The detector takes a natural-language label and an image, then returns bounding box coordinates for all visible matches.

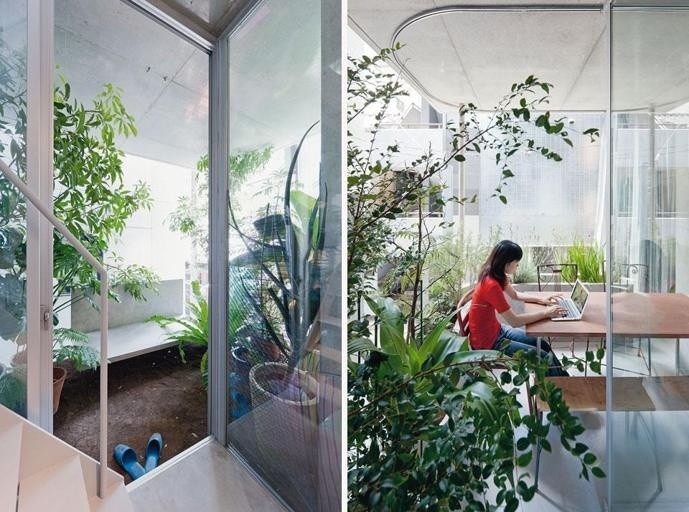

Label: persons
[468,240,570,377]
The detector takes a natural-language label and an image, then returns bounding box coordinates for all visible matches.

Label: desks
[524,291,689,492]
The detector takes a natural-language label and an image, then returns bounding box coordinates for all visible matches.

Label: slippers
[143,431,163,472]
[113,444,144,480]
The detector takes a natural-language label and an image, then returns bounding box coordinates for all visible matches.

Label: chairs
[457,288,533,416]
[536,261,652,376]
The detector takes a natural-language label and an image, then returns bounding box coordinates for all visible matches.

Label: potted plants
[224,118,328,471]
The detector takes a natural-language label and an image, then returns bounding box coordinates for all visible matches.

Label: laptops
[546,278,589,321]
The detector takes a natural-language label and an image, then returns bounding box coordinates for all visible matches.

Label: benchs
[532,376,689,491]
